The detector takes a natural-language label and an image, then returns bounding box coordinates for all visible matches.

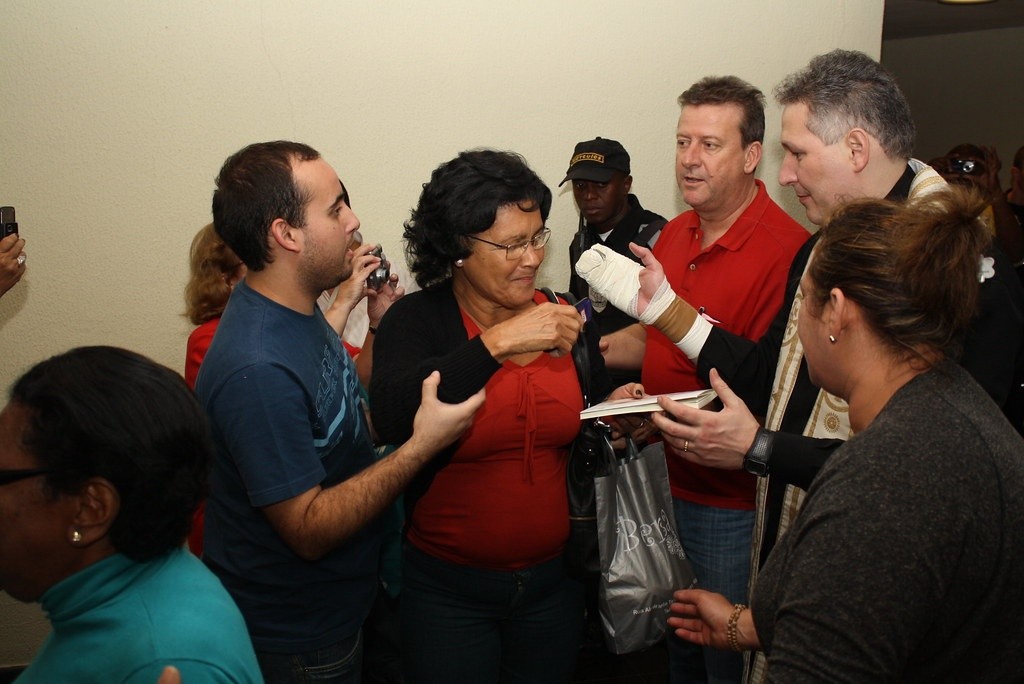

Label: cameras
[951,158,978,175]
[363,244,390,293]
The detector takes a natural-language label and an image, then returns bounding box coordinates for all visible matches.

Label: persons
[368,147,653,683]
[929,143,1024,440]
[668,174,1024,684]
[185,141,487,683]
[0,346,264,683]
[0,204,28,304]
[556,48,990,684]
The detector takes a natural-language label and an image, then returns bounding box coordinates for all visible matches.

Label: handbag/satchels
[594,424,699,654]
[544,288,619,583]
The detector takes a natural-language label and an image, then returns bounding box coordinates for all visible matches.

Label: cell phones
[573,297,592,326]
[0,206,18,242]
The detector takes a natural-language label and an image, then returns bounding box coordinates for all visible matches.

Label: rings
[635,419,646,428]
[684,439,688,451]
[17,255,26,266]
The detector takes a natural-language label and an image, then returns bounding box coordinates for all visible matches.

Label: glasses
[469,226,551,260]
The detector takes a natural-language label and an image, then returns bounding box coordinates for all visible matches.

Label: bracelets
[368,325,377,334]
[727,604,746,652]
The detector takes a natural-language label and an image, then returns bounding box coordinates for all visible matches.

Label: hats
[558,137,631,187]
